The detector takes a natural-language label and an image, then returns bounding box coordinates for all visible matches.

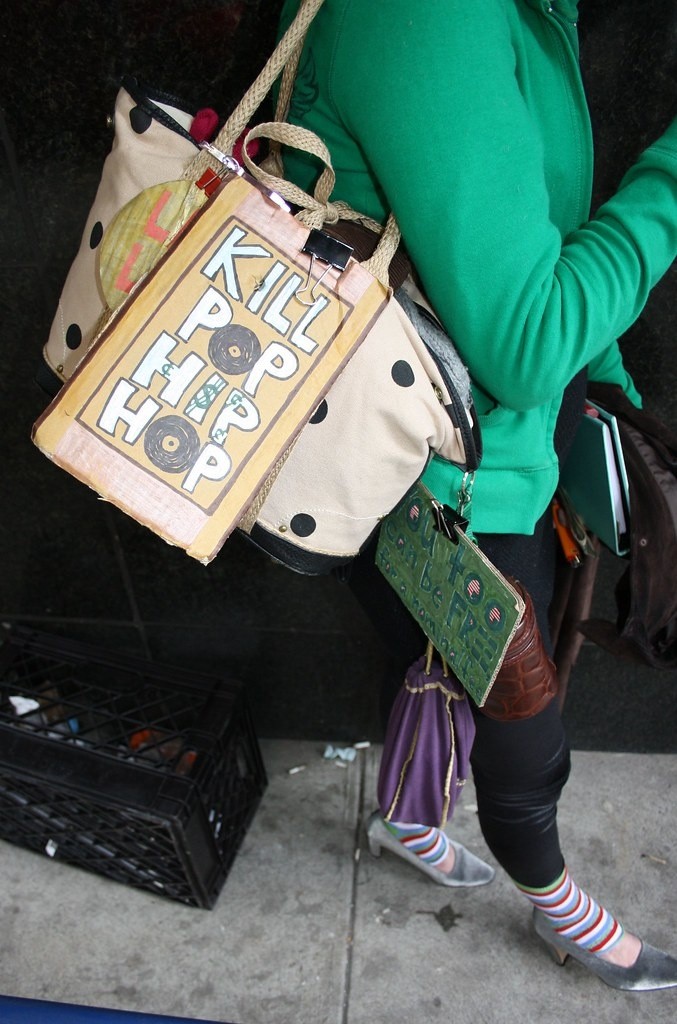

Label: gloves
[187,108,259,169]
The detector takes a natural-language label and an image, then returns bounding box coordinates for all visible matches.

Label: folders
[556,396,637,556]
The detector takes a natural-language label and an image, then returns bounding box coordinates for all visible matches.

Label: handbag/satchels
[43,1,483,577]
[376,636,476,834]
[477,573,560,724]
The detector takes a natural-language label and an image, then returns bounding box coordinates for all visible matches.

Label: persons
[274,0,677,993]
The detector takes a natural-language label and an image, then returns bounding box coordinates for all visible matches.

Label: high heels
[531,905,677,992]
[365,809,497,888]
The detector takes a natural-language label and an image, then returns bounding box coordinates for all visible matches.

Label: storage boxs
[0,623,266,907]
